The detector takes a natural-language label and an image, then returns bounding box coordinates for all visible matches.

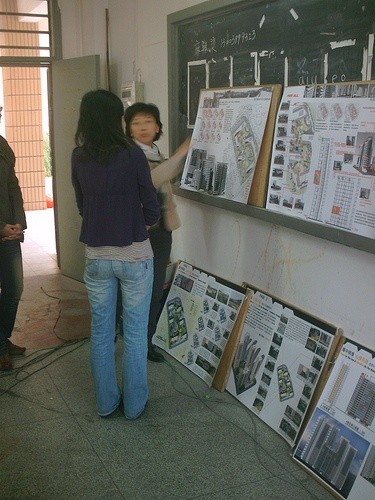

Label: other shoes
[0,350,12,371]
[147,348,165,362]
[5,342,26,355]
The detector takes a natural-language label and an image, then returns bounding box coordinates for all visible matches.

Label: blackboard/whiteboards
[164,1,375,254]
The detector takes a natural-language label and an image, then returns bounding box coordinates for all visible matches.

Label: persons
[71,89,161,420]
[124,102,190,362]
[0,135,27,371]
